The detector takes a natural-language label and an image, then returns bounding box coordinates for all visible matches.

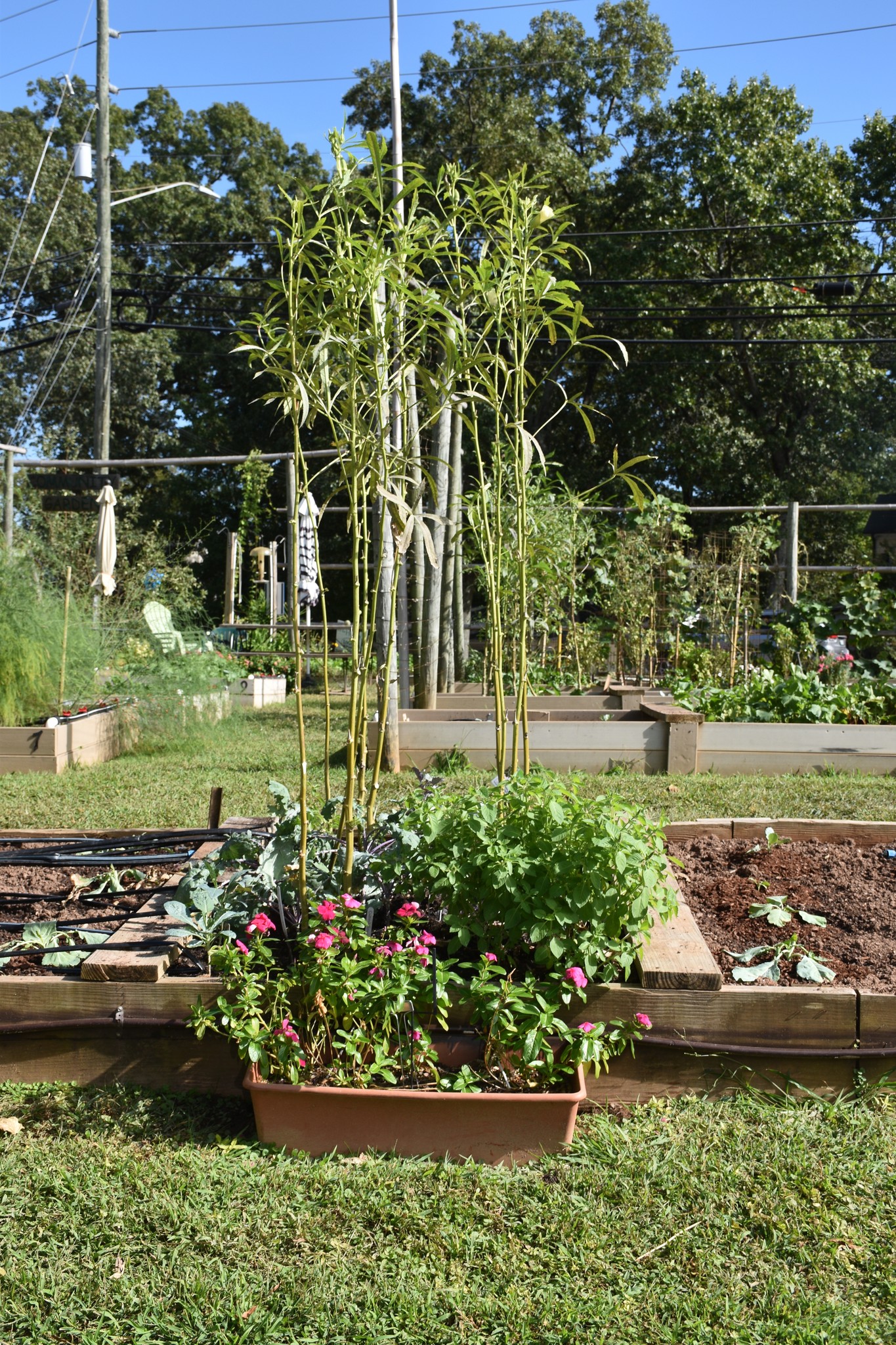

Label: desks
[220,623,372,694]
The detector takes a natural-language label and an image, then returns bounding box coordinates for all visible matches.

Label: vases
[237,1036,596,1157]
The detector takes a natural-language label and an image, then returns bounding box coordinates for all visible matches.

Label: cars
[685,610,850,658]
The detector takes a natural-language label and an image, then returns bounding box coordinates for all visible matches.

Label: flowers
[185,891,649,1094]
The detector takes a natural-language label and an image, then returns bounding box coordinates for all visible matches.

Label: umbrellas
[297,492,320,674]
[90,479,117,623]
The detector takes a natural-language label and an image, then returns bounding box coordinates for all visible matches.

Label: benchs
[231,650,377,659]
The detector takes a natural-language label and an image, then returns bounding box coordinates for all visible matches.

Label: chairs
[145,602,212,662]
